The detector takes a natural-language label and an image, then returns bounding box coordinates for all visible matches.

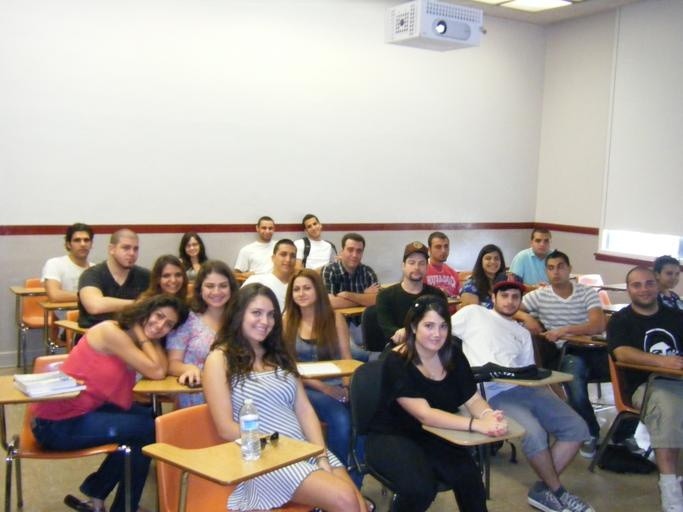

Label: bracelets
[466,416,476,433]
[478,407,494,420]
[136,338,151,350]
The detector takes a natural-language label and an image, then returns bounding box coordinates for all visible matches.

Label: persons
[518,249,612,460]
[509,225,555,287]
[175,231,208,283]
[450,272,595,512]
[279,268,366,492]
[292,214,336,276]
[459,244,524,311]
[71,228,153,351]
[203,283,368,512]
[163,261,238,388]
[374,241,449,350]
[366,294,509,512]
[232,217,279,280]
[605,267,683,512]
[236,239,297,317]
[136,254,195,306]
[317,232,380,347]
[423,231,463,315]
[48,222,93,346]
[47,294,191,511]
[653,255,683,310]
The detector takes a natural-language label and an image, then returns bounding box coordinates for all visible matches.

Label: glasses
[259,431,280,447]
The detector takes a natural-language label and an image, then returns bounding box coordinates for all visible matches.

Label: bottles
[238,399,261,460]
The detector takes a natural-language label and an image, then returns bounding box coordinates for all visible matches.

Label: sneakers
[525,488,571,511]
[579,437,597,457]
[617,438,645,455]
[558,491,595,512]
[657,479,683,512]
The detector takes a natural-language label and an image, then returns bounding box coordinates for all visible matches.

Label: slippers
[63,494,107,512]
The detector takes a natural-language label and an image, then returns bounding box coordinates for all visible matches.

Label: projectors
[386,1,484,52]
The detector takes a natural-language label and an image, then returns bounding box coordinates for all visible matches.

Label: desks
[0,373,84,451]
[38,301,78,355]
[9,283,47,326]
[335,305,370,319]
[54,320,93,351]
[595,281,634,292]
[142,432,325,511]
[490,363,575,389]
[603,303,628,312]
[566,273,579,280]
[295,357,365,382]
[421,409,526,447]
[133,377,205,416]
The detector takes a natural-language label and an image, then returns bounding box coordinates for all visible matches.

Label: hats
[403,242,429,262]
[493,272,528,293]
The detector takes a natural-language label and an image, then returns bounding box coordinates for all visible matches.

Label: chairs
[588,351,656,472]
[349,358,493,509]
[53,303,70,347]
[150,401,316,512]
[17,274,60,368]
[65,307,83,353]
[3,352,133,512]
[532,318,618,402]
